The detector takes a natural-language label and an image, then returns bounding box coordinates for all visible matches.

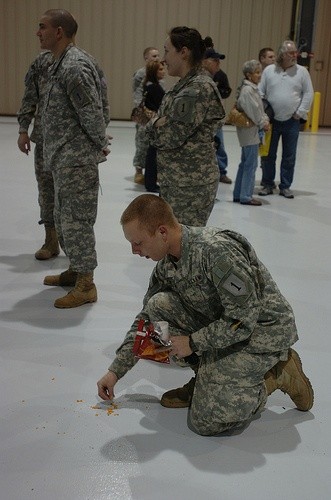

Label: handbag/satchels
[258,122,272,156]
[229,84,254,128]
[130,91,154,126]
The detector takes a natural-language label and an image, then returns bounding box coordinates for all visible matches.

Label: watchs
[188,334,200,352]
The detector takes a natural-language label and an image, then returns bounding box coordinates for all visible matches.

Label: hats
[203,47,225,60]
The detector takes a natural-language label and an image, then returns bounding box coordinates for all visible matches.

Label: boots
[55,272,97,307]
[135,167,144,185]
[35,223,60,259]
[160,362,199,408]
[44,265,78,287]
[263,348,314,411]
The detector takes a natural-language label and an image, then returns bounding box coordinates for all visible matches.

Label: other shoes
[146,184,161,193]
[261,182,276,189]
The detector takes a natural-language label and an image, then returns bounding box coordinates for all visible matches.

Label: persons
[36,10,114,308]
[17,9,111,260]
[97,194,314,436]
[128,26,315,228]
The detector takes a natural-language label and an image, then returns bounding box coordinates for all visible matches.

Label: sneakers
[259,184,273,195]
[279,188,294,198]
[220,176,232,184]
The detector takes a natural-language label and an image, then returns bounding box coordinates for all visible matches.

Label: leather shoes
[233,198,262,206]
